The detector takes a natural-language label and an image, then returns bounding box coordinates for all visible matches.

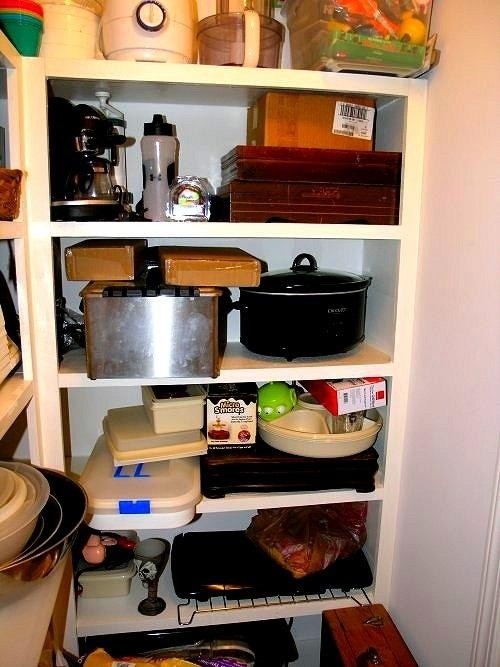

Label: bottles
[140,114,177,221]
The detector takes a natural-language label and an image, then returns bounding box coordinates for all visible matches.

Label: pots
[232,252,374,363]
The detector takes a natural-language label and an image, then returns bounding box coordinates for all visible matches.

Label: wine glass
[134,538,171,616]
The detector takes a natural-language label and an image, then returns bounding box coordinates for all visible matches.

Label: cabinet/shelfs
[0,0,429,667]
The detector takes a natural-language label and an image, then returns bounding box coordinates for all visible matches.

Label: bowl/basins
[257,417,382,458]
[0,0,41,55]
[0,460,89,608]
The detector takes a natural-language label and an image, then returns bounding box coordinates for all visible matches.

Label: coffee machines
[48,98,122,221]
